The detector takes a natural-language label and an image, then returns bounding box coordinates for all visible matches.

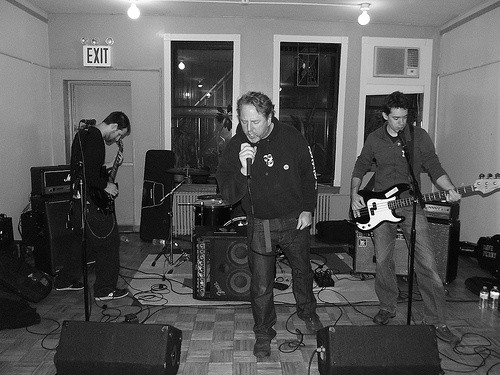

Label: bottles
[490,286,500,310]
[479,285,489,310]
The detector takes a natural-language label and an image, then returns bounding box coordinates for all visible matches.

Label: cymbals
[165,168,211,177]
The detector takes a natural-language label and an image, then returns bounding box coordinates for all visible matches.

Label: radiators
[171,191,331,238]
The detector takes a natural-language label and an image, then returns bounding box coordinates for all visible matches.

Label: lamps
[78,36,113,67]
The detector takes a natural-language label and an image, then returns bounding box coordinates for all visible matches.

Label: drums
[193,199,233,229]
[223,216,247,227]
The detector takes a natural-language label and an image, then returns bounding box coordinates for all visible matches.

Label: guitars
[79,137,124,241]
[351,173,500,234]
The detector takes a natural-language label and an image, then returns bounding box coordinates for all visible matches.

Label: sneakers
[297,311,324,333]
[94,288,130,300]
[55,282,84,290]
[435,325,455,342]
[254,337,271,357]
[373,310,396,324]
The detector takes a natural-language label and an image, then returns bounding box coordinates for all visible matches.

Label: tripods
[150,176,190,267]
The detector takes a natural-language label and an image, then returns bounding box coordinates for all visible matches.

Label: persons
[217,91,323,357]
[55,110,131,301]
[351,91,461,344]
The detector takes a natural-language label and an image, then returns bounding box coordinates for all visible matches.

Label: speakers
[30,199,90,271]
[426,220,460,282]
[54,321,181,375]
[192,236,253,302]
[316,324,443,375]
[353,235,411,277]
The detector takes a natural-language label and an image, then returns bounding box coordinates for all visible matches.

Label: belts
[263,219,272,253]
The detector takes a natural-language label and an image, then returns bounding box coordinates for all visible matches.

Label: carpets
[126,253,380,307]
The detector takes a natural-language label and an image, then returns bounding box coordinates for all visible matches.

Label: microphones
[246,158,253,176]
[397,130,408,148]
[80,119,97,125]
